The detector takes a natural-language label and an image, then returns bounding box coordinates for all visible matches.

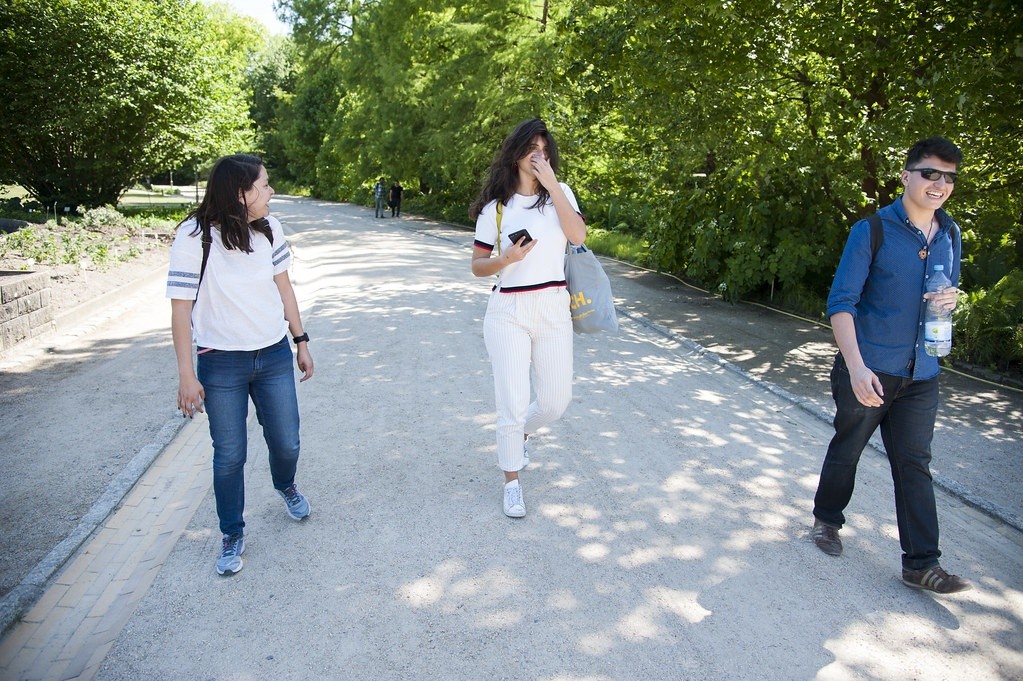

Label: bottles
[924,265,951,357]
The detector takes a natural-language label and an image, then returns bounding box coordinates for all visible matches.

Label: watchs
[293,332,310,344]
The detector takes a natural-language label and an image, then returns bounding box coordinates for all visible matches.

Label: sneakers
[278,484,311,520]
[813,517,843,556]
[216,534,245,574]
[523,436,529,465]
[503,479,526,517]
[902,564,973,594]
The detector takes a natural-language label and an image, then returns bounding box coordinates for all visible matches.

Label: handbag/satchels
[564,241,618,334]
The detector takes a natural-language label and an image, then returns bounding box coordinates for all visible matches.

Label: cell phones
[507,229,532,247]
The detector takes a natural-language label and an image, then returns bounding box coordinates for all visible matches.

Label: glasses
[908,169,959,184]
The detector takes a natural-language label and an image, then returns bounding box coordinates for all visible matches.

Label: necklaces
[910,217,933,260]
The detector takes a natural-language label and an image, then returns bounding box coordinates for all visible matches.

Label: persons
[166,154,314,576]
[812,137,973,594]
[374,177,386,218]
[472,118,586,518]
[390,180,403,217]
